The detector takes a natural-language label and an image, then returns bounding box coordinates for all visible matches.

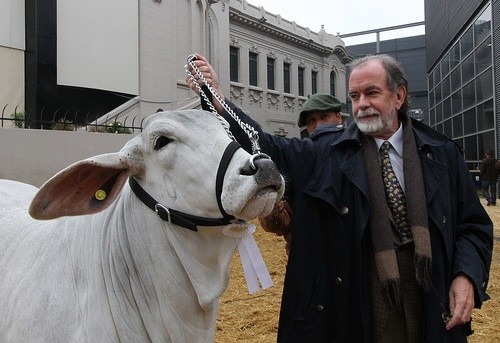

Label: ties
[379,140,413,240]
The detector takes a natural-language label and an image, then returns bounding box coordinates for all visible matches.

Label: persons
[188,54,493,343]
[479,150,498,205]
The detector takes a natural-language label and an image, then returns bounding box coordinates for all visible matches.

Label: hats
[299,94,342,128]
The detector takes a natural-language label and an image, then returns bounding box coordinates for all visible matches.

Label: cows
[0,108,286,343]
